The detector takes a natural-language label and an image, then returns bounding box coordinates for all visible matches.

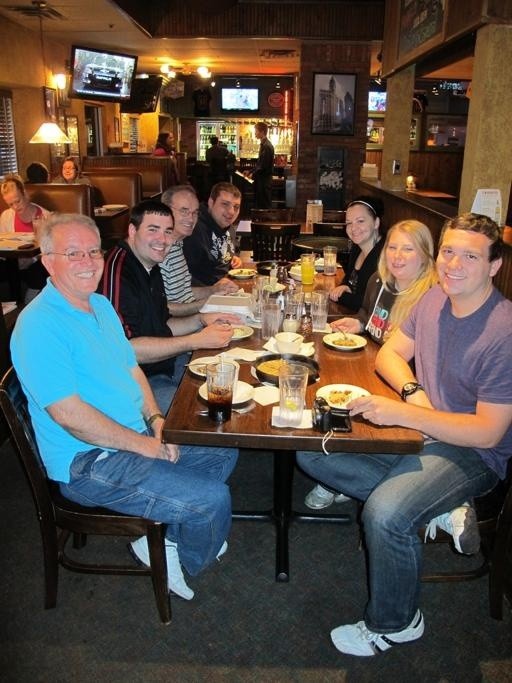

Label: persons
[156,183,239,314]
[295,213,511,656]
[52,156,95,207]
[303,221,440,509]
[96,198,245,419]
[9,213,240,600]
[242,122,274,210]
[0,173,51,314]
[26,162,48,184]
[329,196,388,312]
[151,131,181,186]
[183,183,241,287]
[206,137,235,184]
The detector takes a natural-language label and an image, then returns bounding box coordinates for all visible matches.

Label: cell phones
[329,404,352,434]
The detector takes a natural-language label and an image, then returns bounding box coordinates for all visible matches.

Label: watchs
[401,382,422,402]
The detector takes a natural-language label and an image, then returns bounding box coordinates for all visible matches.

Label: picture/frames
[317,145,346,208]
[311,72,356,135]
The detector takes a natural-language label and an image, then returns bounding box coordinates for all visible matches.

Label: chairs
[312,222,349,237]
[239,220,301,261]
[0,364,175,626]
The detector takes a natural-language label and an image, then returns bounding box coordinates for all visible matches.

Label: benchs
[0,165,167,248]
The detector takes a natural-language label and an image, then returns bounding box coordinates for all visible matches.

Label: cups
[311,291,328,328]
[324,246,338,275]
[251,285,268,324]
[261,298,281,338]
[278,364,309,423]
[301,254,315,284]
[208,362,236,423]
[267,332,316,360]
[282,290,305,333]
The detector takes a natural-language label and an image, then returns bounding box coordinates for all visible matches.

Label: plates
[253,354,323,386]
[230,326,254,341]
[198,380,254,405]
[324,333,366,351]
[230,269,256,279]
[315,384,369,410]
[104,204,128,210]
[190,356,240,378]
[19,233,34,241]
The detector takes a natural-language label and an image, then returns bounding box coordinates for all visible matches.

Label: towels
[249,382,282,405]
[271,409,313,428]
[215,346,271,362]
[264,336,278,353]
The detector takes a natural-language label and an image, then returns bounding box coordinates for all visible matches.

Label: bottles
[271,263,287,283]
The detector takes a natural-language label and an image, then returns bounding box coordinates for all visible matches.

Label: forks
[194,402,255,417]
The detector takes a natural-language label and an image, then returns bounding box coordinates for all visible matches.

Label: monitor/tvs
[118,76,163,114]
[65,45,139,104]
[220,85,260,113]
[367,89,387,114]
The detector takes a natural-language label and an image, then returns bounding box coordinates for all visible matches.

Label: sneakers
[212,540,229,563]
[420,501,481,560]
[329,609,424,658]
[335,492,351,505]
[129,528,194,602]
[305,484,334,510]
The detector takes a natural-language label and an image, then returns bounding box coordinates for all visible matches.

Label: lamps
[27,13,74,145]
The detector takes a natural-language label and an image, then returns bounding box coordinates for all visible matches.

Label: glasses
[62,167,76,170]
[169,209,201,219]
[47,249,105,262]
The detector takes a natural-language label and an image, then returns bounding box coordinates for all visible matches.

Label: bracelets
[146,414,163,425]
